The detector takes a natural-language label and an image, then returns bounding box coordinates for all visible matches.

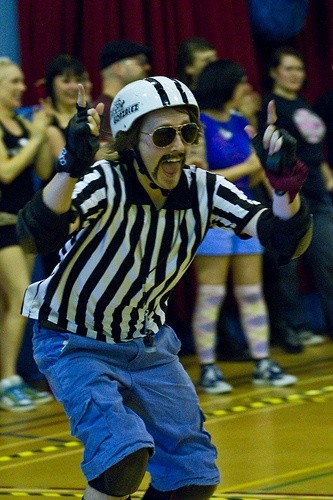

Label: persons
[260,51,333,353]
[187,58,298,394]
[178,37,261,363]
[33,52,93,186]
[15,76,313,500]
[0,55,54,412]
[94,41,152,161]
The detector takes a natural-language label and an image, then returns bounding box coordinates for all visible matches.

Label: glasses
[138,122,200,147]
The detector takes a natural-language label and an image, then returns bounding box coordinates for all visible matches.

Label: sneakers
[196,367,233,393]
[1,382,34,411]
[252,360,298,387]
[20,384,54,405]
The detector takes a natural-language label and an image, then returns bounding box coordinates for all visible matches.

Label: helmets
[111,75,201,140]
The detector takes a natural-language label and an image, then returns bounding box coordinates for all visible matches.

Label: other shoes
[299,331,326,346]
[275,323,303,352]
